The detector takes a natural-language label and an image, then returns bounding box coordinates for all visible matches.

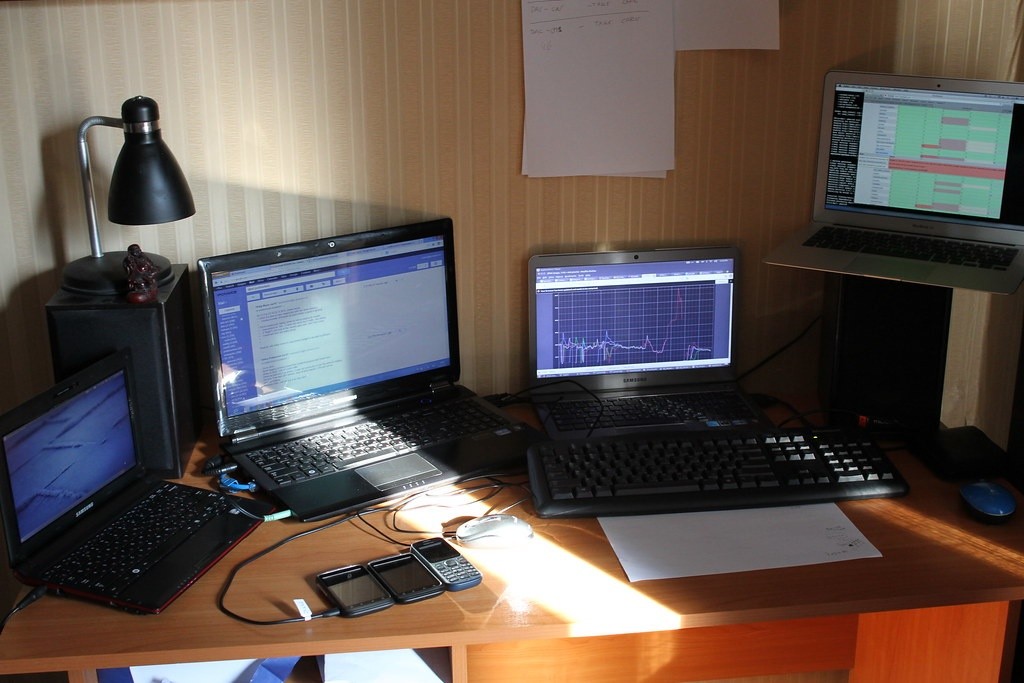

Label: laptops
[0,347,276,617]
[525,246,776,443]
[198,217,550,523]
[759,69,1024,296]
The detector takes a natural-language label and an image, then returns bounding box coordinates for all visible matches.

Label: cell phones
[316,564,395,617]
[368,553,444,605]
[411,537,482,592]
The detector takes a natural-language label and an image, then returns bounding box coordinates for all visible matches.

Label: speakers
[43,264,205,480]
[826,274,952,439]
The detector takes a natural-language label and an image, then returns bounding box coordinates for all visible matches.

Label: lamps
[58,95,196,300]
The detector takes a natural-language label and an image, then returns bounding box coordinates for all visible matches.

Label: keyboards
[526,425,910,518]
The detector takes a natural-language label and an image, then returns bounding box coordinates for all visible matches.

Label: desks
[0,406,1024,683]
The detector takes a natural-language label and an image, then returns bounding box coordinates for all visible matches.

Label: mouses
[455,513,536,545]
[961,480,1017,525]
